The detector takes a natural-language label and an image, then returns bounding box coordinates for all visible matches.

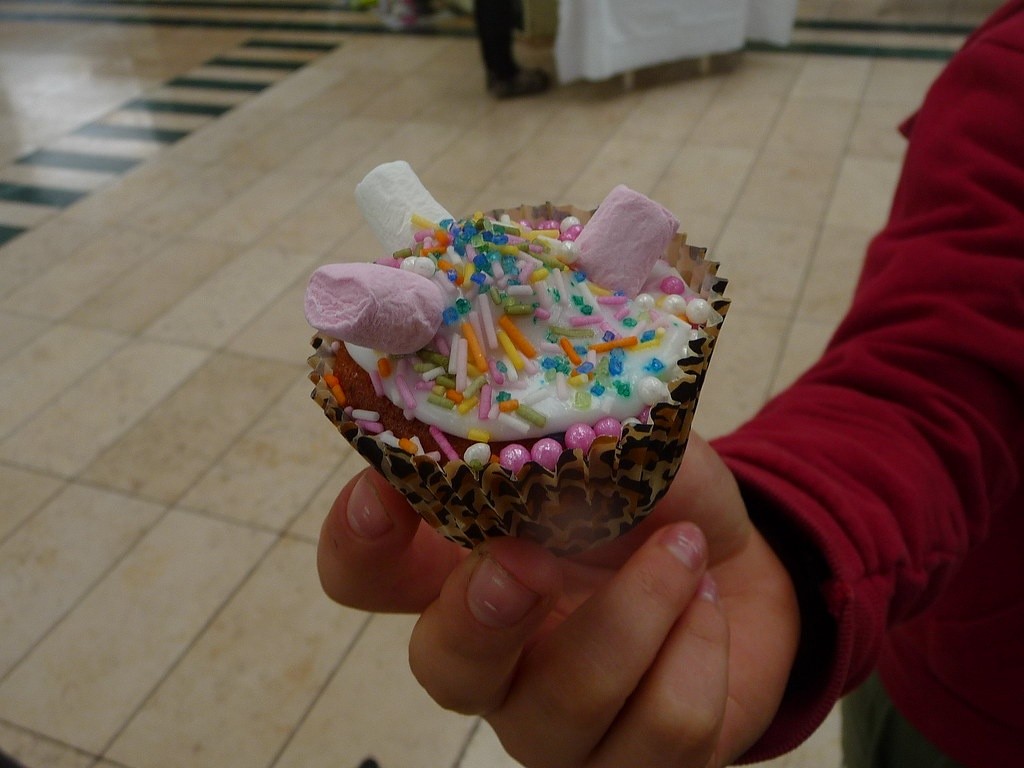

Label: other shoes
[484,68,548,97]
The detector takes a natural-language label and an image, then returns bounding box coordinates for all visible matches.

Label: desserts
[300,159,733,567]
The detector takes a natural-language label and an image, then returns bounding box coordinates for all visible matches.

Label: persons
[316,1,1024,768]
